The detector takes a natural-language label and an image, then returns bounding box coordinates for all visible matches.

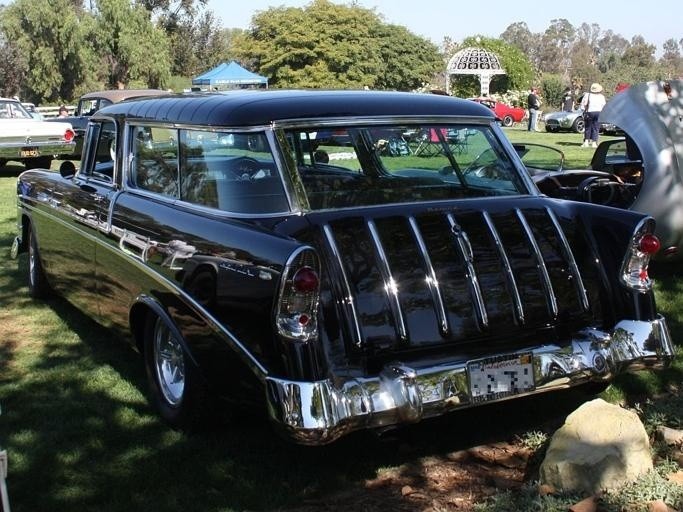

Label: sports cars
[379,77,682,269]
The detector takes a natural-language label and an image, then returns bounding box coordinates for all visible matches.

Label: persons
[580,82,606,148]
[58,107,68,119]
[528,87,540,132]
[561,87,575,113]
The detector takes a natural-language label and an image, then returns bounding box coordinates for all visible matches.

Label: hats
[590,83,602,93]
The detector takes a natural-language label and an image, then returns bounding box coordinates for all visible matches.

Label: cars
[10,86,681,461]
[544,137,644,181]
[0,95,77,173]
